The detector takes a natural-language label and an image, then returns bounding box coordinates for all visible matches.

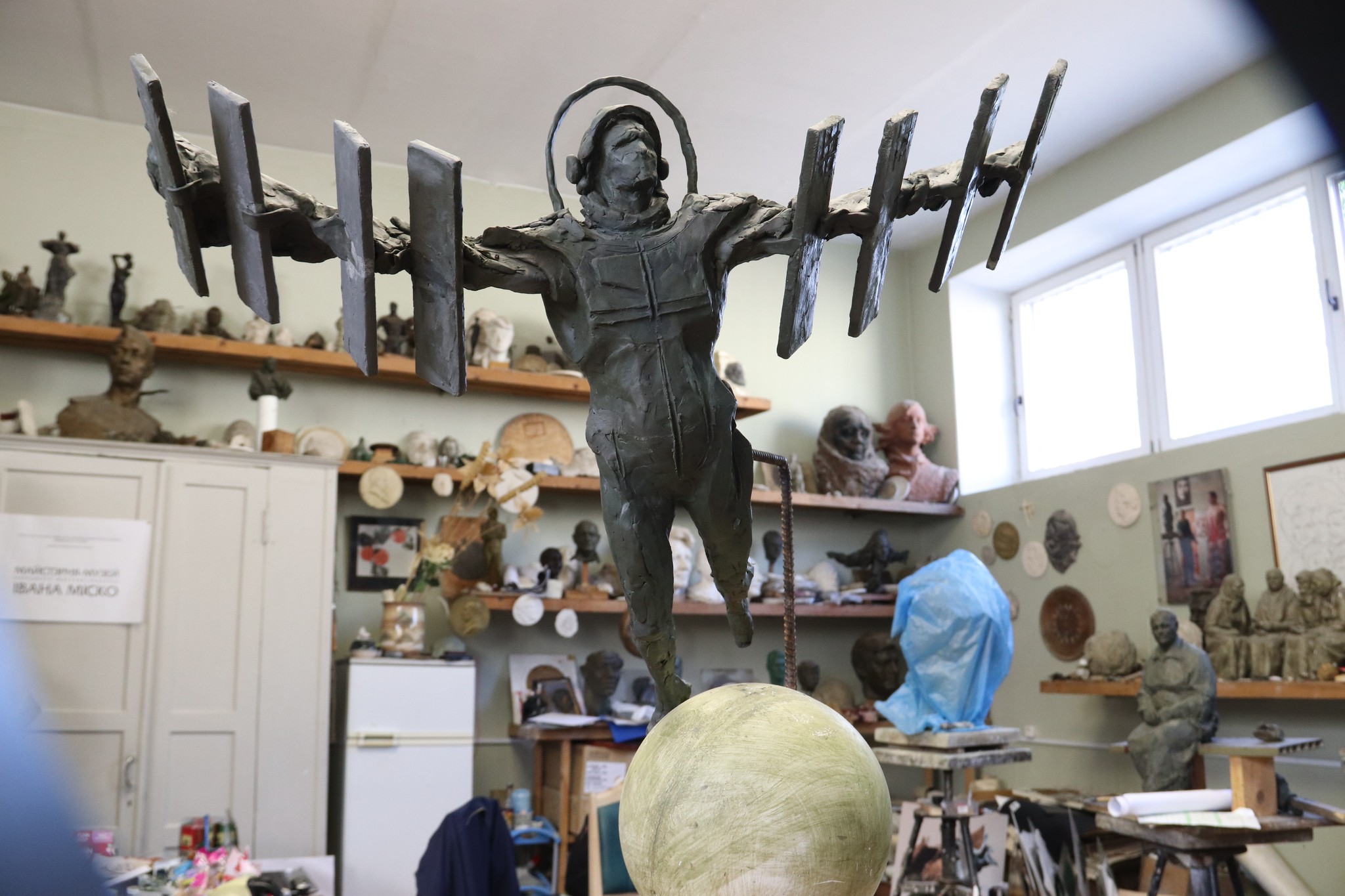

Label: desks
[508,676,1345,896]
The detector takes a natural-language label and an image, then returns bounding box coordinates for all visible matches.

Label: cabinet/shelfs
[0,312,965,616]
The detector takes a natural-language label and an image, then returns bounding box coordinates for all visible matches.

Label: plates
[293,426,350,462]
[495,413,574,469]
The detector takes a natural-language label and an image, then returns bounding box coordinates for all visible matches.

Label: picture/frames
[347,515,424,590]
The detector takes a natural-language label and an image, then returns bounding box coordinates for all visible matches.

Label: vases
[380,602,427,660]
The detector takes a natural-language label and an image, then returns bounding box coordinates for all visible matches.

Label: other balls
[618,682,892,896]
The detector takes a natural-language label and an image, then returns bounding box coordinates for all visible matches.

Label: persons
[668,526,693,601]
[851,631,904,722]
[1204,575,1249,681]
[1126,611,1220,793]
[578,651,623,717]
[572,521,600,562]
[376,301,414,354]
[479,506,509,563]
[56,324,161,443]
[812,406,888,496]
[871,399,960,502]
[143,103,1029,737]
[686,546,724,604]
[1249,567,1345,681]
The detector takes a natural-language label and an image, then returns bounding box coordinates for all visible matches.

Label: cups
[381,602,426,659]
[512,788,532,838]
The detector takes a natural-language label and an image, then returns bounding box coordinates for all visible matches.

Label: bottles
[180,814,238,862]
